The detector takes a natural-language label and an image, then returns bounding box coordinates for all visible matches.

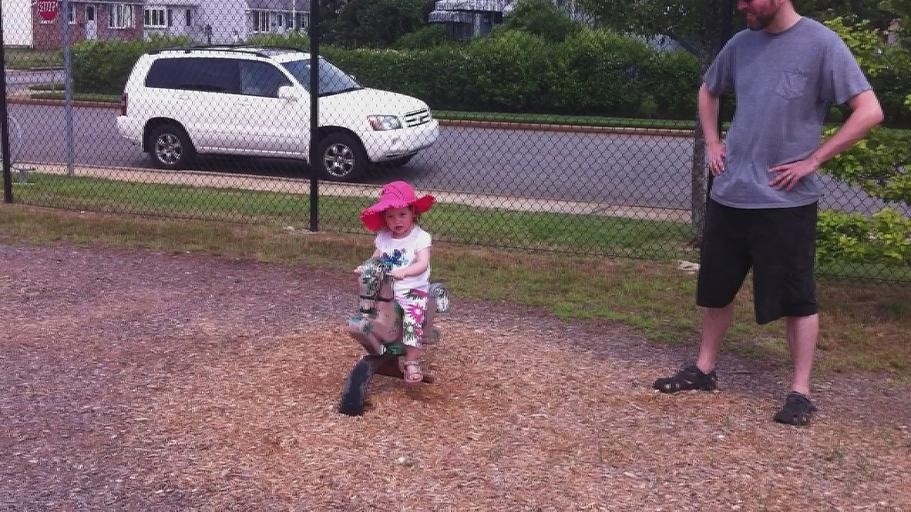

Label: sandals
[773,391,818,425]
[402,357,424,385]
[651,364,717,394]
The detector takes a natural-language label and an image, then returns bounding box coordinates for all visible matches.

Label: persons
[354,181,436,384]
[651,0,885,425]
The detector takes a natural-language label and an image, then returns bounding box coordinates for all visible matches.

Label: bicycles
[0,113,24,172]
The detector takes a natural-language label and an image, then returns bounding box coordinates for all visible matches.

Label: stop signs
[36,0,58,23]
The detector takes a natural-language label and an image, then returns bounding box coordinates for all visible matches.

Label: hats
[360,180,437,233]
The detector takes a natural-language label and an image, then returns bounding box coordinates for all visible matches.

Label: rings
[787,175,792,182]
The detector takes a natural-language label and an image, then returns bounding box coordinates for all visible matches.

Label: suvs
[116,39,438,183]
